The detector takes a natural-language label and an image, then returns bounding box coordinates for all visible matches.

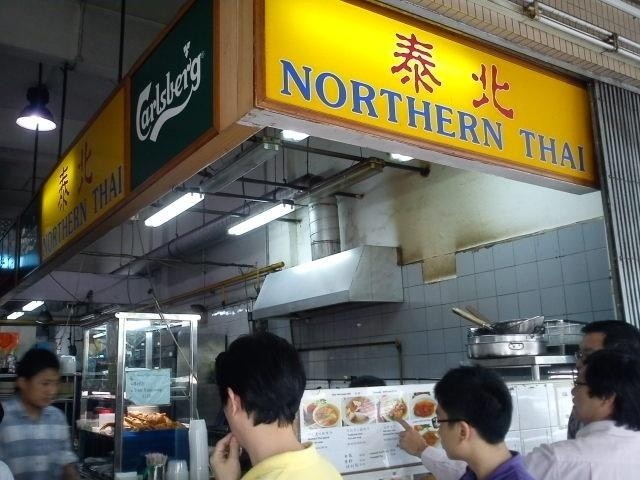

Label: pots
[466,317,586,359]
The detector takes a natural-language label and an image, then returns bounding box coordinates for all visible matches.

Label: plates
[74,310,202,480]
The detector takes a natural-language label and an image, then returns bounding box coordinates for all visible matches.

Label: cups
[432,417,465,429]
[574,351,589,361]
[573,380,589,387]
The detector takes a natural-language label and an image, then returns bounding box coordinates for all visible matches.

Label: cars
[167,459,188,480]
[188,419,210,479]
[142,463,166,479]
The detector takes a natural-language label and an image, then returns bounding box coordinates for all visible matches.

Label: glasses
[15,84,57,133]
[142,136,387,235]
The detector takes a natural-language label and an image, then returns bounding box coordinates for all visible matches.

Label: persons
[0,348,82,480]
[575,320,640,361]
[0,401,14,480]
[567,362,590,438]
[348,377,385,387]
[397,351,640,479]
[432,363,534,480]
[208,329,342,480]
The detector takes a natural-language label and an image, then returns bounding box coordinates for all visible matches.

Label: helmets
[305,394,437,429]
